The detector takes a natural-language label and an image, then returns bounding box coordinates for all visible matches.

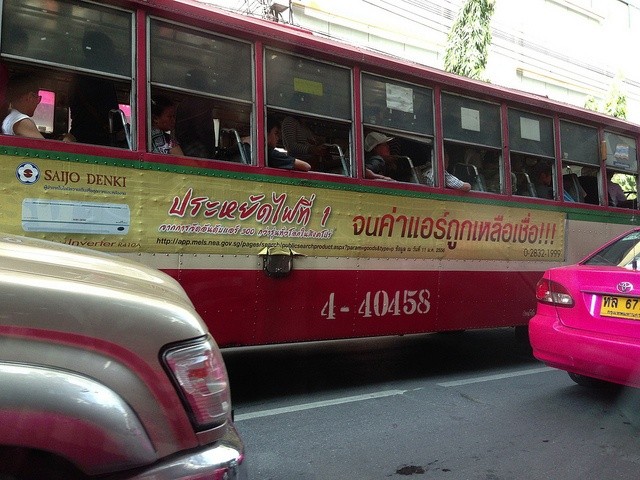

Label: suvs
[1,232,243,480]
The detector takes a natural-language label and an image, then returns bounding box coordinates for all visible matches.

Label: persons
[282,93,330,172]
[268,118,312,171]
[1,25,29,115]
[607,169,628,208]
[2,78,77,142]
[414,141,471,192]
[483,150,517,195]
[151,96,184,155]
[69,31,126,147]
[534,165,565,201]
[238,126,250,146]
[365,131,400,181]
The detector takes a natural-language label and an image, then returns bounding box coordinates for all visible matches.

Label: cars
[527,227,639,403]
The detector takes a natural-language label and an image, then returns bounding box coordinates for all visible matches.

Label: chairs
[517,171,537,197]
[109,108,131,149]
[394,155,423,184]
[325,143,349,176]
[220,127,249,163]
[458,163,485,191]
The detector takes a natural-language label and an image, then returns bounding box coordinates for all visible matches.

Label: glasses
[34,95,41,104]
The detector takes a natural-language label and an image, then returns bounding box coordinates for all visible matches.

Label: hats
[365,132,395,153]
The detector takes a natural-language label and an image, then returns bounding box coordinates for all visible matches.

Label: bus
[1,1,640,355]
